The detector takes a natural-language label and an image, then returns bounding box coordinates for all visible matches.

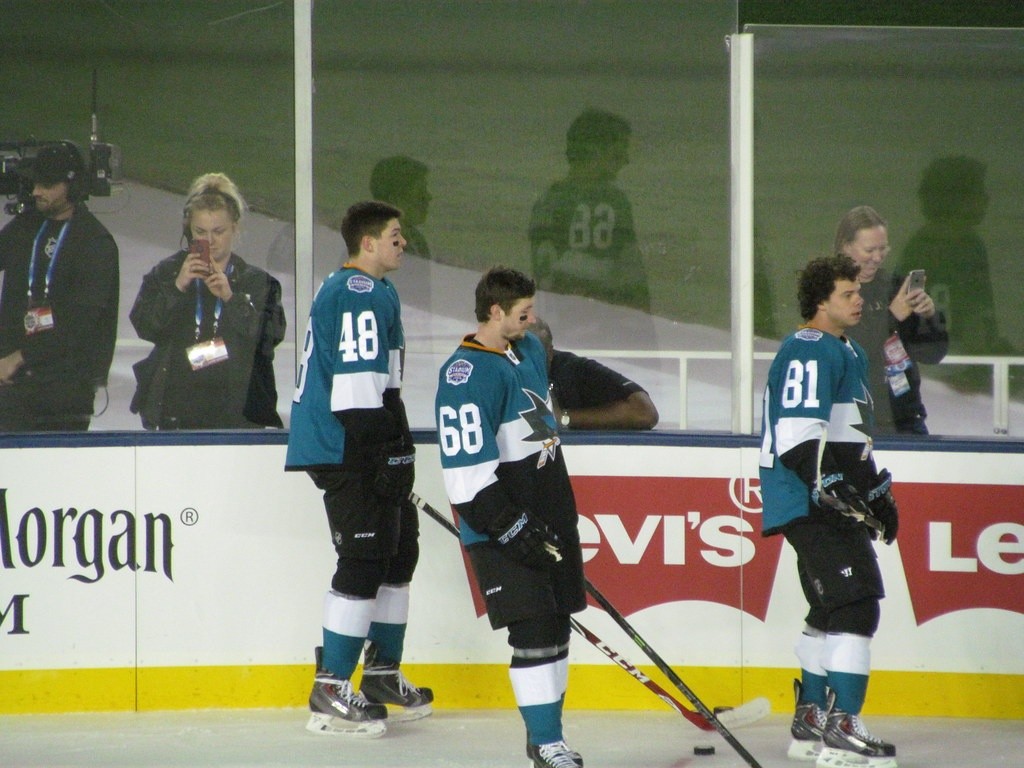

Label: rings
[190,268,192,272]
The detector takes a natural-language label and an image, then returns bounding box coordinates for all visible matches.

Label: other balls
[694,744,715,755]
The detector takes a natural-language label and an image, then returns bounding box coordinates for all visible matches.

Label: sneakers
[819,691,898,767]
[359,638,434,724]
[533,741,584,768]
[307,646,387,737]
[526,733,581,766]
[786,679,828,760]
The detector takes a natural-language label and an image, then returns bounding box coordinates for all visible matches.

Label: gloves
[868,468,898,545]
[376,446,415,507]
[809,471,883,530]
[511,524,570,575]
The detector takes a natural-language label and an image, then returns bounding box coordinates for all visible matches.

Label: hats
[16,141,84,182]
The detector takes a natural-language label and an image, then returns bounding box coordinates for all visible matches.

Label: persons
[284,200,434,739]
[761,255,899,768]
[527,111,661,372]
[893,155,1024,392]
[0,141,120,431]
[527,317,660,431]
[129,172,287,431]
[834,206,948,436]
[434,265,587,768]
[369,157,432,313]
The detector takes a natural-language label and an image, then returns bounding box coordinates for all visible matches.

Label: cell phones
[908,269,926,294]
[191,240,211,276]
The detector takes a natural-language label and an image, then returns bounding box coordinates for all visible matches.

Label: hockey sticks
[812,428,883,533]
[405,489,771,729]
[581,577,762,768]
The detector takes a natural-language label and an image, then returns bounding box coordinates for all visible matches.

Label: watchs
[561,411,570,428]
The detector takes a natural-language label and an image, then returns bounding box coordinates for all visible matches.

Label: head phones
[60,140,91,203]
